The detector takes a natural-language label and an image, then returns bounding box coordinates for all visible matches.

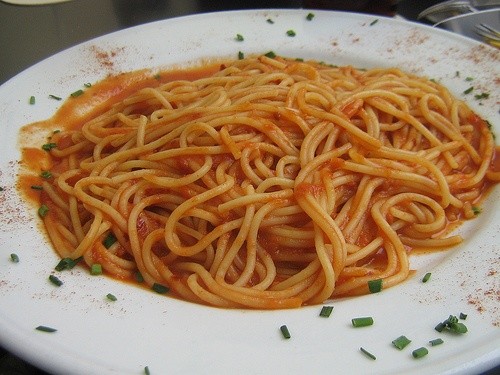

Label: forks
[473,22,500,41]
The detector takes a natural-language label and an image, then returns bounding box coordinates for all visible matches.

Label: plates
[0,9,500,374]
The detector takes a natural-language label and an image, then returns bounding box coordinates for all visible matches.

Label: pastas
[39,57,499,311]
